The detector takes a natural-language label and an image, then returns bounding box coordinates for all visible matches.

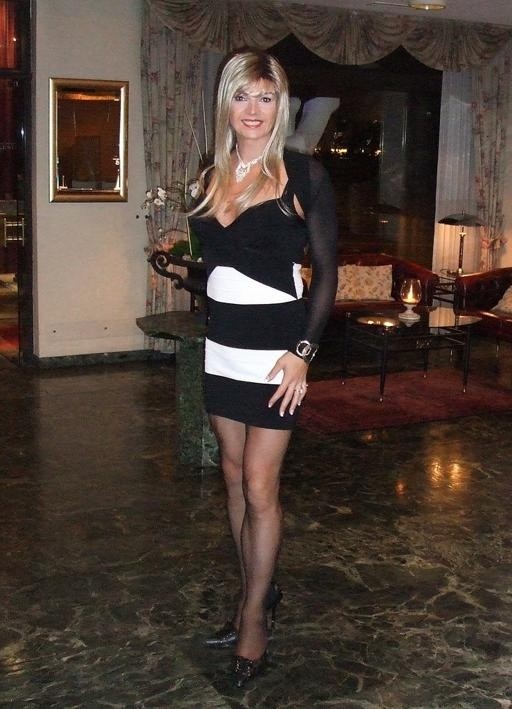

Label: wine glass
[398,276,422,321]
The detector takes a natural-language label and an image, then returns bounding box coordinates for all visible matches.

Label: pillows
[303,262,396,303]
[491,285,511,312]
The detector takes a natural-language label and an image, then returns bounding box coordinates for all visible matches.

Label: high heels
[203,581,284,688]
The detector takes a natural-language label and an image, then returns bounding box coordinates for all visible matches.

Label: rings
[293,389,303,393]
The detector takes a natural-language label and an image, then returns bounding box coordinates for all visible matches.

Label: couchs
[302,250,441,356]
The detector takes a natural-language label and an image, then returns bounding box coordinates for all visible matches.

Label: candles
[402,298,417,303]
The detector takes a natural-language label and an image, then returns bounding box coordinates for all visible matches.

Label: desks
[138,311,222,468]
[431,270,502,359]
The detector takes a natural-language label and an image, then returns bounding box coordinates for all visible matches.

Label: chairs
[454,264,510,353]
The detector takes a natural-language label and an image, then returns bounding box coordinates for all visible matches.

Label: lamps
[437,207,485,274]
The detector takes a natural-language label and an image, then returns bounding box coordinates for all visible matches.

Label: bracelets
[292,338,320,362]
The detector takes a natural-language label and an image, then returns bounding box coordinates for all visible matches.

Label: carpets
[291,367,511,438]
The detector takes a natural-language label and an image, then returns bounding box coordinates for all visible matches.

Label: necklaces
[234,142,272,185]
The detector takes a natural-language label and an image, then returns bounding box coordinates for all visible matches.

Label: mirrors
[47,75,130,202]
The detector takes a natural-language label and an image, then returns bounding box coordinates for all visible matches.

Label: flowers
[141,173,198,220]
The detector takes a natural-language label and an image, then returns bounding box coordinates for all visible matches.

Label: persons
[180,51,340,688]
